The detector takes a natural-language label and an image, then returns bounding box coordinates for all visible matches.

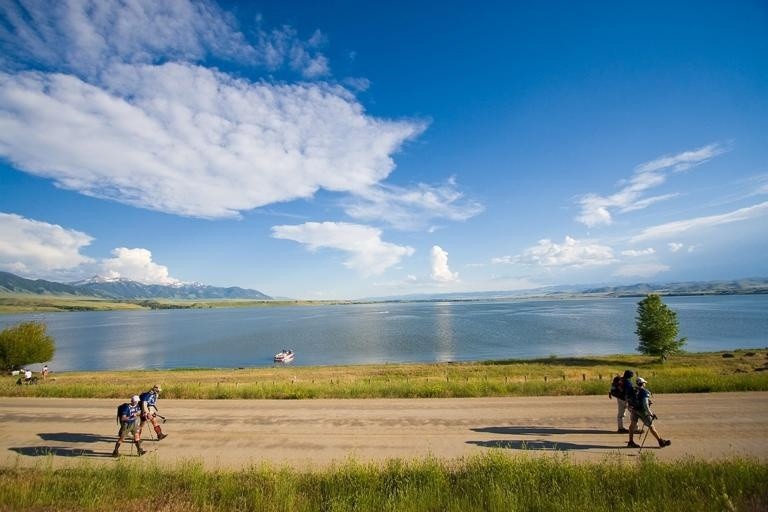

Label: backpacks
[628,387,646,411]
[611,376,630,398]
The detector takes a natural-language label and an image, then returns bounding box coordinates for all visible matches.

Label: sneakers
[618,428,670,448]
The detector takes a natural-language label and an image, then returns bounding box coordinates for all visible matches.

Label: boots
[112,432,168,457]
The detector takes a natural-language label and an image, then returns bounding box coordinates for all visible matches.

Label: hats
[152,384,162,391]
[132,395,141,402]
[635,377,648,385]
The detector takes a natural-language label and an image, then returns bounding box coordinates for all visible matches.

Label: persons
[627,377,671,447]
[42,364,48,379]
[25,369,32,381]
[132,384,168,443]
[112,395,146,457]
[617,371,645,434]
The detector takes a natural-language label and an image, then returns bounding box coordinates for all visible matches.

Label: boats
[274,349,295,364]
